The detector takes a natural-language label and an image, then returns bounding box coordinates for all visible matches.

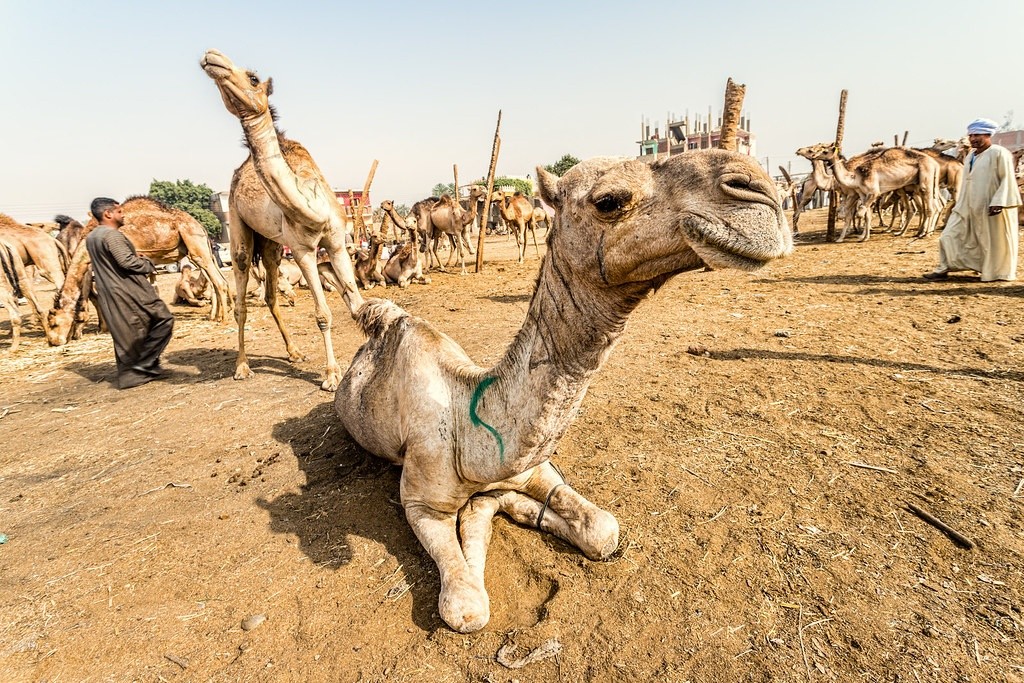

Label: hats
[967,118,1000,137]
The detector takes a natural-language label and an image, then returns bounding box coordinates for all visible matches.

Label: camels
[200,47,365,393]
[792,137,973,244]
[0,186,550,347]
[337,148,796,634]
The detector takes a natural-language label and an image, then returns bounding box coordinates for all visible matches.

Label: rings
[994,209,997,213]
[997,210,1001,213]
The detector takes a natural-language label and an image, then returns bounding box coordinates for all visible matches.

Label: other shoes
[132,364,164,375]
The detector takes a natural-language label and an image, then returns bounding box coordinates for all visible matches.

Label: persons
[923,119,1022,283]
[85,197,174,389]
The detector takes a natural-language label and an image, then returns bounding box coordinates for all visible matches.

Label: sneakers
[922,272,948,280]
